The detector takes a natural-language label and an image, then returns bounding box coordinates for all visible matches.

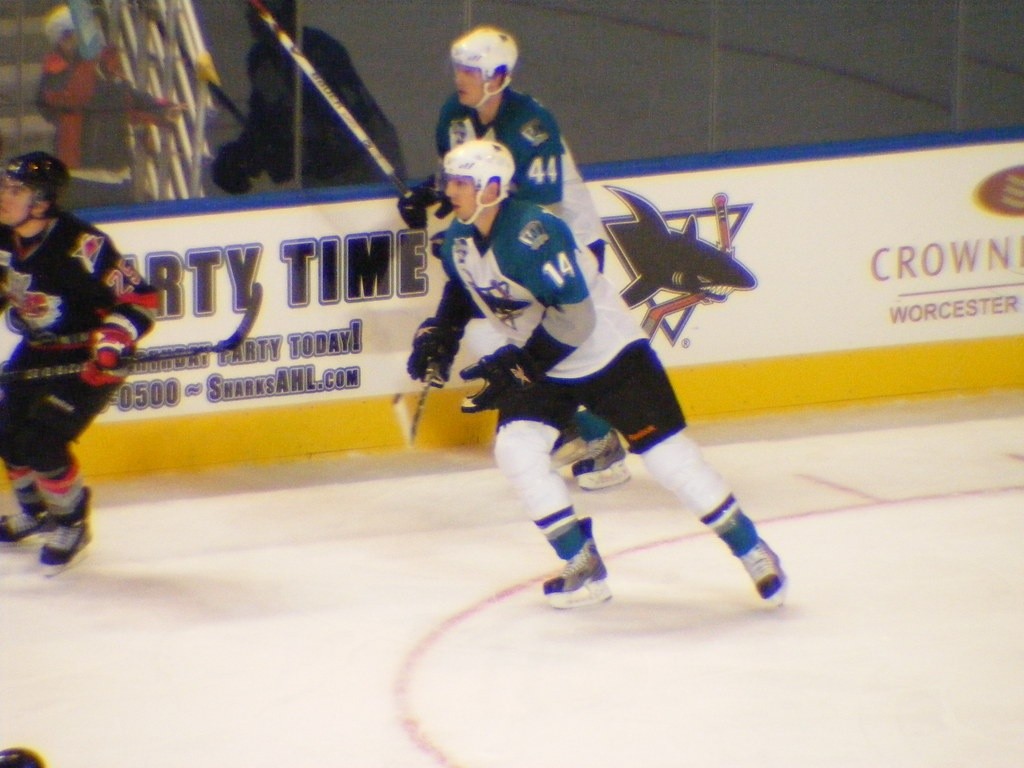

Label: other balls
[0,747,46,768]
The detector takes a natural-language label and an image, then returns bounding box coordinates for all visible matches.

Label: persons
[406,140,785,600]
[34,4,190,208]
[0,150,158,564]
[397,28,626,478]
[211,0,406,196]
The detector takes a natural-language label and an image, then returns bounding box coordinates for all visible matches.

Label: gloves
[210,141,255,194]
[406,316,462,381]
[82,325,137,388]
[458,344,547,414]
[398,181,455,236]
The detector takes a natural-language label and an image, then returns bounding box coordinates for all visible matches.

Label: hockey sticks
[391,360,441,449]
[251,2,413,200]
[1,280,267,388]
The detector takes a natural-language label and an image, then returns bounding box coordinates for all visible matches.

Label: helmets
[443,138,517,201]
[43,8,77,47]
[451,26,518,81]
[6,151,69,206]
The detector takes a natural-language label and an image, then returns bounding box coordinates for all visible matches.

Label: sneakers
[543,516,614,609]
[0,511,53,548]
[572,426,632,489]
[738,537,789,607]
[40,487,94,576]
